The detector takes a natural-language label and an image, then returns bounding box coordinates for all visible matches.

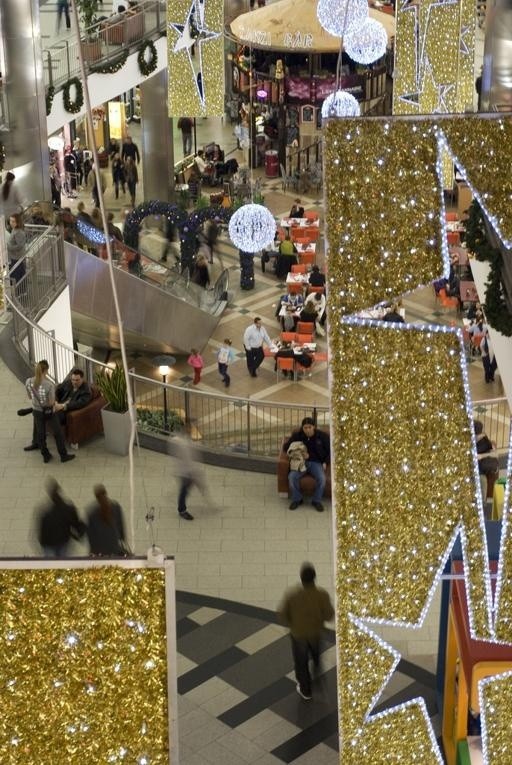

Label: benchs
[100,4,146,46]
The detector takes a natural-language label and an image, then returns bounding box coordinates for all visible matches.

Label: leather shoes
[179,511,194,520]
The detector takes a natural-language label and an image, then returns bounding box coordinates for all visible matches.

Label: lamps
[339,15,393,66]
[227,1,281,260]
[319,1,365,121]
[312,1,371,35]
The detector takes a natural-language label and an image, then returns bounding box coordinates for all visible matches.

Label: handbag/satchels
[42,405,53,419]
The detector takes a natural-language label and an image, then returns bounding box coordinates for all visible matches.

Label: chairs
[43,386,108,448]
[434,209,490,358]
[275,425,332,500]
[259,206,329,387]
[181,137,324,209]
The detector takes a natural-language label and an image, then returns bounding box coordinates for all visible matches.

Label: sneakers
[311,499,324,512]
[23,442,38,451]
[484,375,496,385]
[16,406,33,417]
[289,499,304,511]
[44,454,53,463]
[60,454,76,462]
[296,683,313,701]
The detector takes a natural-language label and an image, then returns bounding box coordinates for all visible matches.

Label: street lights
[126,353,144,405]
[152,355,177,432]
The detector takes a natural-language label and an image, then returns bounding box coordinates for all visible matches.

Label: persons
[94,0,138,27]
[280,562,334,700]
[3,52,511,559]
[55,0,71,31]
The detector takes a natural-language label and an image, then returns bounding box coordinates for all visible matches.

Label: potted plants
[94,360,137,457]
[75,0,104,65]
